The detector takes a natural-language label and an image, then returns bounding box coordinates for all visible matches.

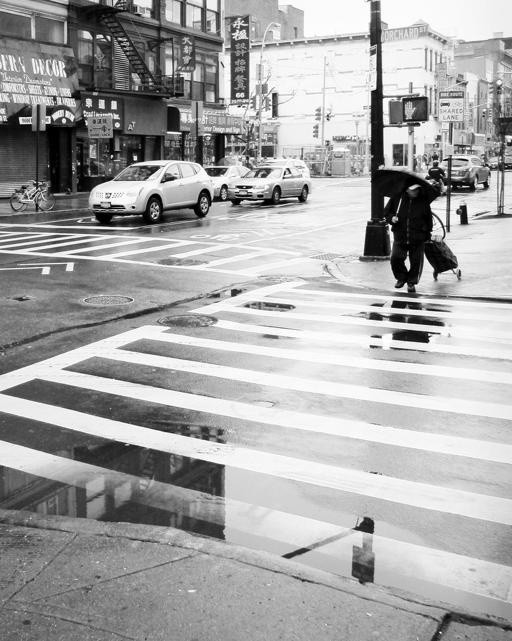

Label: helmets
[433,160,439,165]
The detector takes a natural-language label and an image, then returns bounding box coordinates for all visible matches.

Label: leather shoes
[395,280,406,288]
[407,282,415,292]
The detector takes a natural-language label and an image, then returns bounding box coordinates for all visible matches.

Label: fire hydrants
[457,201,468,225]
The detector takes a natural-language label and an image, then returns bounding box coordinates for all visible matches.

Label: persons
[381,179,435,294]
[427,161,447,195]
[431,152,439,161]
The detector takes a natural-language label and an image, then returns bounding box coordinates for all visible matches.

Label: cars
[228,159,312,205]
[88,160,214,224]
[439,154,492,192]
[484,155,512,169]
[204,166,255,203]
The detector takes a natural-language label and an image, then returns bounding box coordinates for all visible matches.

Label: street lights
[320,55,328,144]
[258,22,280,157]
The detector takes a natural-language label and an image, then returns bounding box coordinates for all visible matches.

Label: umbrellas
[369,166,441,205]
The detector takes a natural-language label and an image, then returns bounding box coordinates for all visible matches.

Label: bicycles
[9,181,57,212]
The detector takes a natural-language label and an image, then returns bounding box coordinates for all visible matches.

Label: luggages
[424,242,458,273]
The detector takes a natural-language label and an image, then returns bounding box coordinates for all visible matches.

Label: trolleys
[424,237,462,281]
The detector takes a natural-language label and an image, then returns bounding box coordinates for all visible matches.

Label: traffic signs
[438,90,466,123]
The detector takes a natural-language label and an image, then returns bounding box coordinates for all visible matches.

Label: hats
[406,180,421,190]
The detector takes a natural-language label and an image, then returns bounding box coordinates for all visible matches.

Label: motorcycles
[426,170,444,195]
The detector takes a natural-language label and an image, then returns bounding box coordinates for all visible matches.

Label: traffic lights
[326,115,329,121]
[251,95,256,111]
[483,109,486,119]
[496,79,503,98]
[313,124,319,138]
[316,108,320,120]
[399,96,431,120]
[265,97,271,113]
